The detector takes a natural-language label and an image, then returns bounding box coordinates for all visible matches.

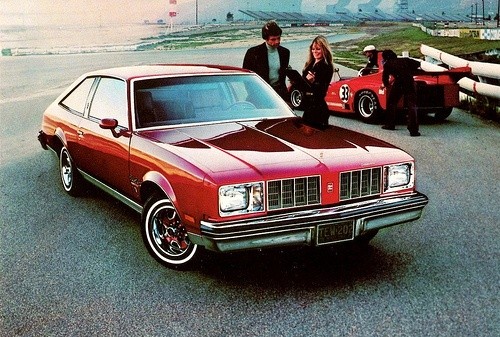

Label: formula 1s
[286,60,472,123]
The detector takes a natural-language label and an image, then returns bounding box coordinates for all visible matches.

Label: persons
[242,21,294,111]
[359,45,384,76]
[290,35,334,126]
[381,49,422,137]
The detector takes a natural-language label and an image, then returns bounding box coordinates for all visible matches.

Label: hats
[363,45,375,52]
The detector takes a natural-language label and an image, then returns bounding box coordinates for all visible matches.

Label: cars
[37,59,430,271]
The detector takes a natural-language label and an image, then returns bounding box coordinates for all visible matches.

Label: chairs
[136,91,157,124]
[188,88,223,118]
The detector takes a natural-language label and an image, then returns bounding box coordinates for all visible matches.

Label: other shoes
[382,126,395,130]
[410,132,420,136]
